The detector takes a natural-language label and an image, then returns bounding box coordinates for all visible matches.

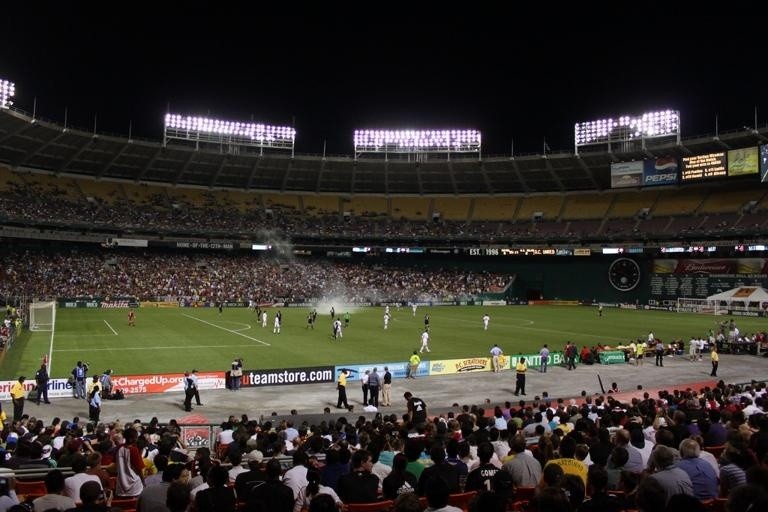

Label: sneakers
[514,392,527,396]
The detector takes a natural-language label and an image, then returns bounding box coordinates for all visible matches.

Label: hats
[247,449,264,464]
[42,444,53,458]
[67,437,84,448]
[543,463,564,479]
[19,376,26,381]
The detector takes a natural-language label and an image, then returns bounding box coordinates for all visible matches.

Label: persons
[0,168,767,512]
